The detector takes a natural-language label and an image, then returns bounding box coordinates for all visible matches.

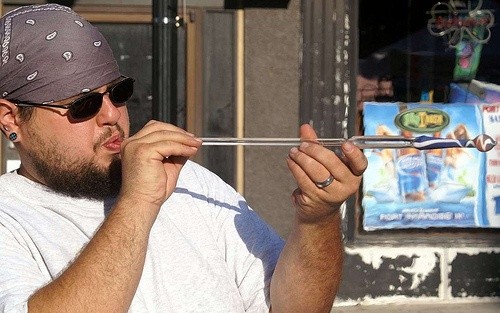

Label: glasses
[5,74,136,122]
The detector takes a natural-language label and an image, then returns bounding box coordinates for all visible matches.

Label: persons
[0,4,368,313]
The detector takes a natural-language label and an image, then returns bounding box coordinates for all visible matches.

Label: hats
[0,4,121,107]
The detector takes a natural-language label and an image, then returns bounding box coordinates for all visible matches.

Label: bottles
[398,103,426,202]
[378,126,396,179]
[427,130,443,188]
[446,125,474,182]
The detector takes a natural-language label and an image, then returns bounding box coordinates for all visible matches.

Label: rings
[316,173,334,188]
